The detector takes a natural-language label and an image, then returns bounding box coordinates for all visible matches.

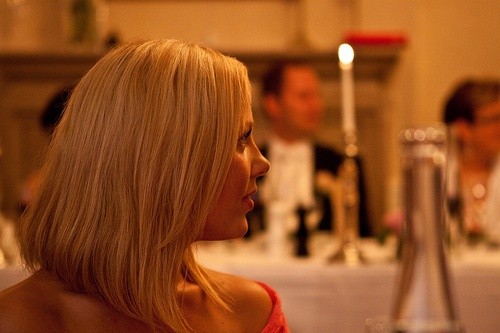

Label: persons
[245,58,372,258]
[438,76,500,257]
[12,82,74,217]
[0,39,290,332]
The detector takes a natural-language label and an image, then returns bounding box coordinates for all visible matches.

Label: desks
[0,222,500,333]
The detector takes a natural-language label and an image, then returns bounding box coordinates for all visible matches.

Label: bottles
[390,122,465,332]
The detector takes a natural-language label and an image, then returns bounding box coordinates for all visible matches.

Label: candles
[336,42,357,137]
